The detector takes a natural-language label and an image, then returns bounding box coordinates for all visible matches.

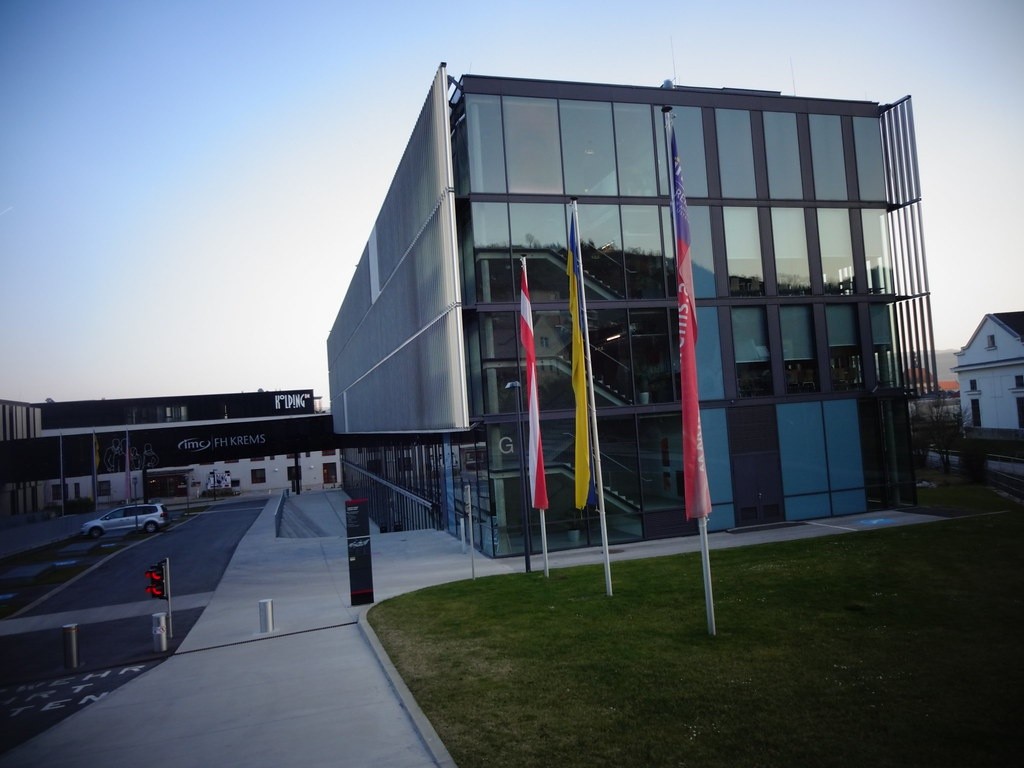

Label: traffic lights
[145,565,165,600]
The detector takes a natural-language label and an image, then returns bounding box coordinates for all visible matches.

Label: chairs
[785,368,850,391]
[730,275,760,297]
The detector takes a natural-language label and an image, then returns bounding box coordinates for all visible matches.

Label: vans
[80,502,170,538]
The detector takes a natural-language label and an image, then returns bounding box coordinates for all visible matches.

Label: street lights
[212,470,218,502]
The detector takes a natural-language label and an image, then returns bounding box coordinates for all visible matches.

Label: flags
[671,127,711,518]
[124,433,130,500]
[92,434,100,499]
[520,270,548,509]
[567,215,597,510]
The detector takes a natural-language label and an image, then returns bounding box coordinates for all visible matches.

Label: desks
[747,283,762,293]
[736,284,747,292]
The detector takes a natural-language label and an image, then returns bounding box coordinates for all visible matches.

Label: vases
[638,392,649,405]
